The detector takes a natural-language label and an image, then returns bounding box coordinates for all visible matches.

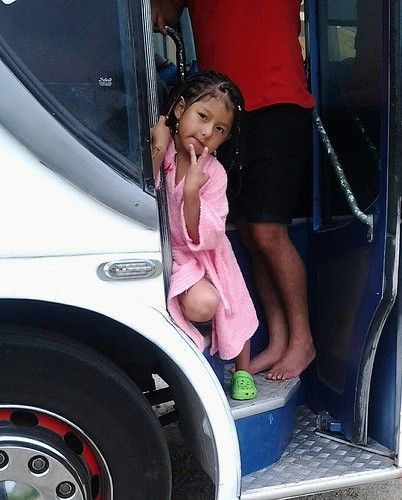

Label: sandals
[231,370,257,400]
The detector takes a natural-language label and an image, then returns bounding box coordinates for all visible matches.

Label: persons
[150,0,316,381]
[150,72,259,400]
